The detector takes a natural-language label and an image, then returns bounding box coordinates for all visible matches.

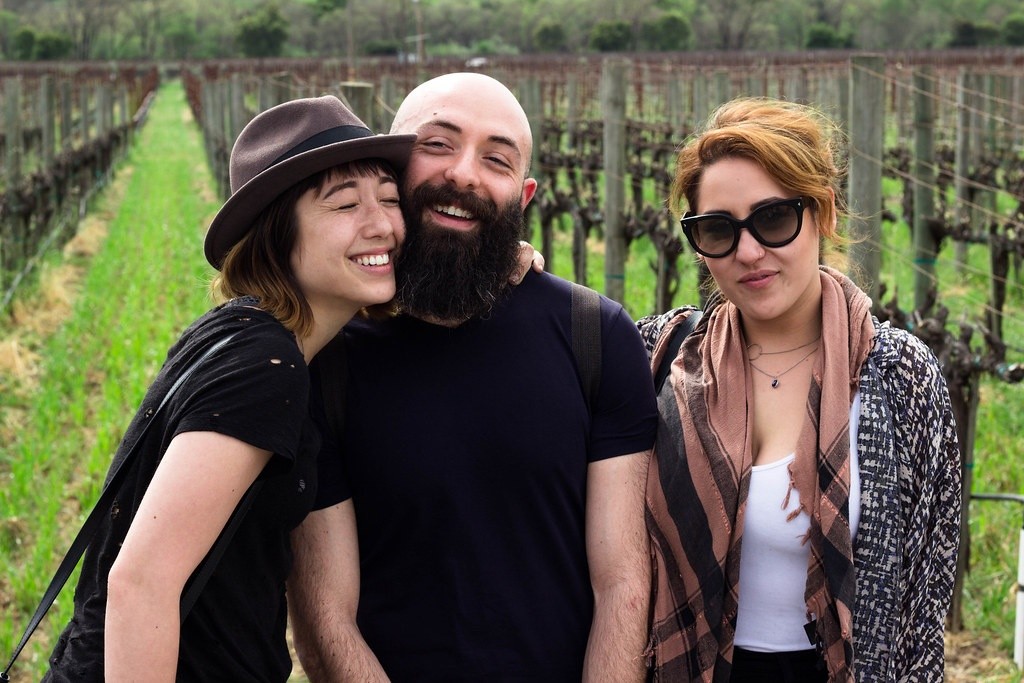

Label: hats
[202,93,418,270]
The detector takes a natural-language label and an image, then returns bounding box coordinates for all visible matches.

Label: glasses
[679,195,810,258]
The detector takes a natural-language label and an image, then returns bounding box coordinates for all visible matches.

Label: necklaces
[746,332,821,388]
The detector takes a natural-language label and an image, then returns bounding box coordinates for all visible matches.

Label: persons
[41,93,418,683]
[286,72,660,683]
[509,97,962,683]
